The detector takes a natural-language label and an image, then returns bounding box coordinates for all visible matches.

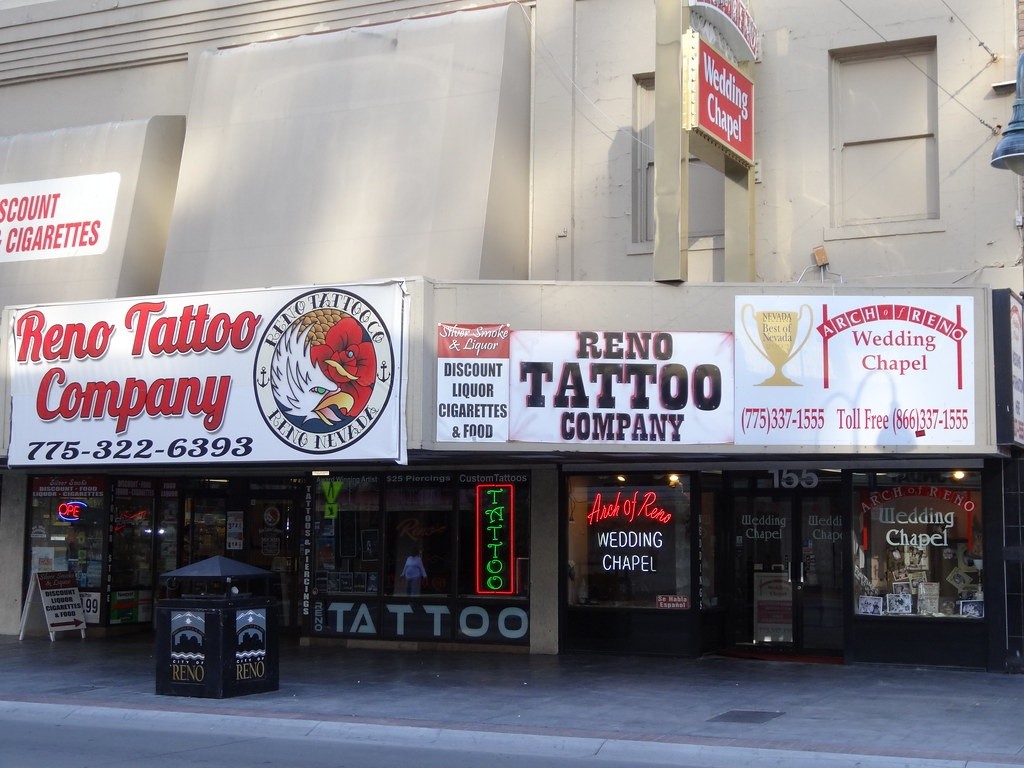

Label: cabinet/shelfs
[32,490,297,623]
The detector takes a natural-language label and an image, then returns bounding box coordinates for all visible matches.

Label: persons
[399,547,427,595]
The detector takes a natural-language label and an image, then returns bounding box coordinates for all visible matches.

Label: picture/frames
[858,541,984,617]
